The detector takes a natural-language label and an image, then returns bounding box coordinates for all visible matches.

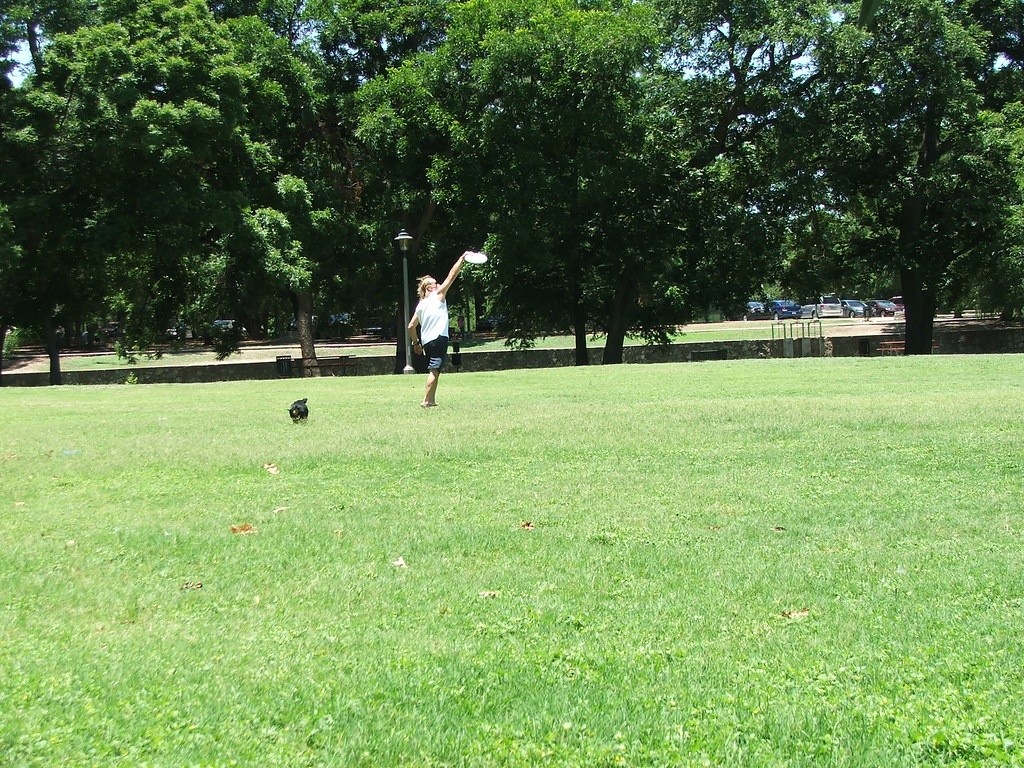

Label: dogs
[287,397,309,424]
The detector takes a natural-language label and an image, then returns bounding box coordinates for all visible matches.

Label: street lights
[393,228,416,374]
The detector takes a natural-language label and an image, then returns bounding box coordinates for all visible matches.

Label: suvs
[799,294,844,319]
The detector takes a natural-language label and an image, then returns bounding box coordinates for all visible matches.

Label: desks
[292,354,356,377]
[878,340,936,356]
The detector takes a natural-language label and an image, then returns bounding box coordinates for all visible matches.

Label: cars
[762,299,803,320]
[726,301,774,321]
[840,299,873,318]
[213,319,249,341]
[865,299,900,318]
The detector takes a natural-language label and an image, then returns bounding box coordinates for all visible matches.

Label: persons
[408,250,474,409]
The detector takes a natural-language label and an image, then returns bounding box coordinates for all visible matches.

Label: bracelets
[411,339,419,346]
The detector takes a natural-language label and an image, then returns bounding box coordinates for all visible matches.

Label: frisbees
[464,252,488,264]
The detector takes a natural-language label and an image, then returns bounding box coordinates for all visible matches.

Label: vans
[889,296,905,318]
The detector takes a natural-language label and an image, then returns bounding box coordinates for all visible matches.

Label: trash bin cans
[276,355,291,378]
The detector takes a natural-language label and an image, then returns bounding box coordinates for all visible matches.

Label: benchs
[291,362,365,368]
[875,346,939,351]
